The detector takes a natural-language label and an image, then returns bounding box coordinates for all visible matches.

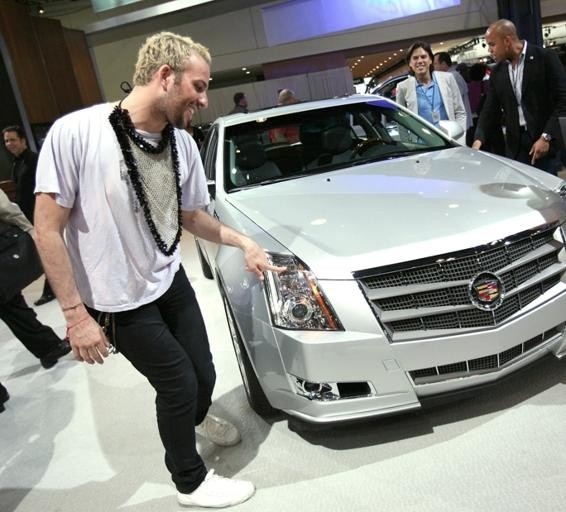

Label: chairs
[232,124,365,185]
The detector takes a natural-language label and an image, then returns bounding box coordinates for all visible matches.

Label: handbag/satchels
[0,220,45,305]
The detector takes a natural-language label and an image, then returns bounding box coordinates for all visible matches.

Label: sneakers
[39,337,71,370]
[33,277,56,306]
[195,413,242,449]
[0,383,9,414]
[175,468,257,510]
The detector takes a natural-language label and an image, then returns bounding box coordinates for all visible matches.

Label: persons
[28,29,290,510]
[465,63,506,156]
[226,91,265,149]
[433,51,475,150]
[1,124,55,306]
[269,89,307,145]
[0,187,72,415]
[471,18,566,178]
[395,41,468,152]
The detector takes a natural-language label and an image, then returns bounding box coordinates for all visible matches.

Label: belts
[517,124,528,133]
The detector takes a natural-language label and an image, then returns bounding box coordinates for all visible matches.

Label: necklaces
[109,93,186,257]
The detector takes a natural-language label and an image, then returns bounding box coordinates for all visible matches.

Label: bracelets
[65,315,90,339]
[61,302,83,313]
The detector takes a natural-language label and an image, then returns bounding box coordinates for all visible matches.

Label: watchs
[541,133,553,142]
